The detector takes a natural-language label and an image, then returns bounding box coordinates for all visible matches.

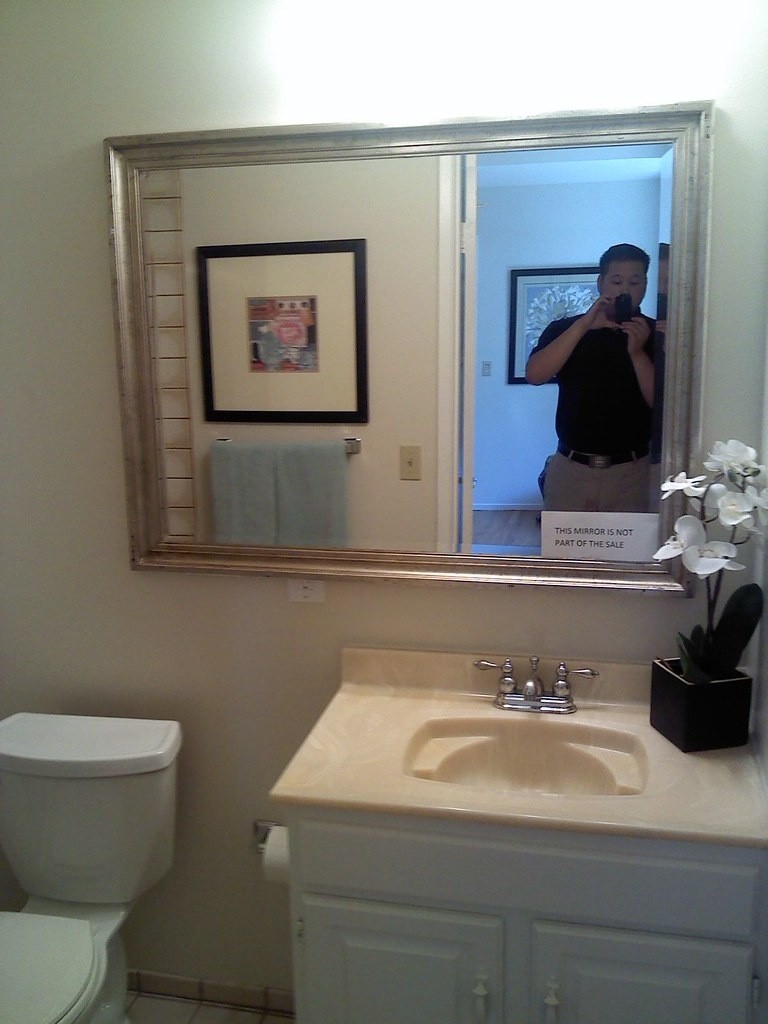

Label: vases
[651,661,754,753]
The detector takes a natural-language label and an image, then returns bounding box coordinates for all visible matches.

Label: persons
[656,243,670,369]
[525,243,657,512]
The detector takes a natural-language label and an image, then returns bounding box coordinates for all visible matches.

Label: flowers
[654,440,767,682]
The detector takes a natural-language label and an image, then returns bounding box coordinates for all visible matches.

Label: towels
[208,437,348,549]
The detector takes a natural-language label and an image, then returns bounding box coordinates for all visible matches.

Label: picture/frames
[506,267,602,385]
[192,237,370,424]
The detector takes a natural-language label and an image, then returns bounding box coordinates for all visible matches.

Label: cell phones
[614,294,632,324]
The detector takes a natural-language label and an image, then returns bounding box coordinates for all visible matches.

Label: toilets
[1,713,185,1023]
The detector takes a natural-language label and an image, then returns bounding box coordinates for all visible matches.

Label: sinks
[400,713,651,798]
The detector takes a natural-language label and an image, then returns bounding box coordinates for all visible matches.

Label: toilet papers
[262,824,292,879]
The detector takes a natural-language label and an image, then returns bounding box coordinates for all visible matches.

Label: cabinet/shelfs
[281,804,768,1023]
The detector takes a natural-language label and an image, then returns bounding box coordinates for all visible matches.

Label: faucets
[522,655,545,712]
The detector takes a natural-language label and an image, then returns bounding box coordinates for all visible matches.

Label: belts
[558,442,647,469]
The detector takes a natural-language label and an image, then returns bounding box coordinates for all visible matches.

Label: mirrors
[107,98,714,598]
